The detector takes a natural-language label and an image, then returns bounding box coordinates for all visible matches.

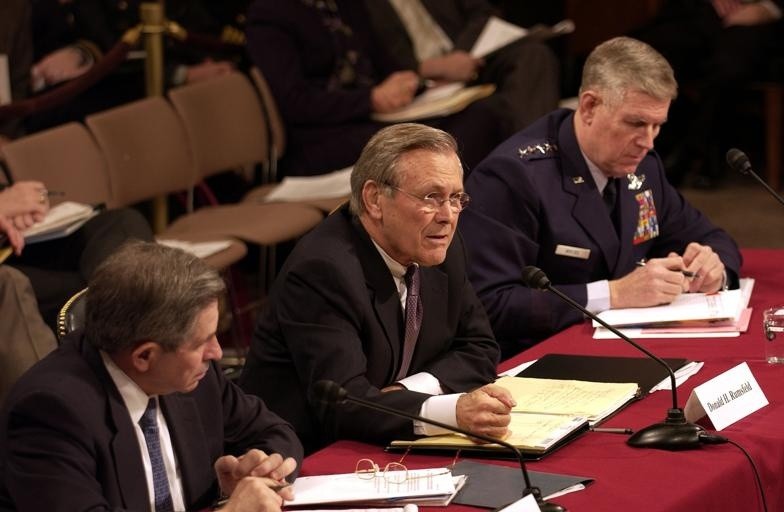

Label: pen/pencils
[589,426,633,434]
[211,482,289,509]
[0,183,67,197]
[633,257,702,278]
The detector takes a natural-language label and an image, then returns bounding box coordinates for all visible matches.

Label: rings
[38,193,45,206]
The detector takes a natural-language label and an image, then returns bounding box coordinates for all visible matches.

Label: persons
[241,120,514,447]
[0,240,304,512]
[458,37,741,358]
[25,1,784,193]
[0,178,61,398]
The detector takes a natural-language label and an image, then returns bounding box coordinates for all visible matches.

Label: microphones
[727,149,784,206]
[309,380,567,512]
[522,266,708,450]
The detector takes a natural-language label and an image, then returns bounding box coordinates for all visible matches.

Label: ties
[603,175,617,215]
[395,264,424,388]
[138,397,175,512]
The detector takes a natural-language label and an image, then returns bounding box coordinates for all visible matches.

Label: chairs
[86,94,324,295]
[2,121,249,358]
[168,73,349,217]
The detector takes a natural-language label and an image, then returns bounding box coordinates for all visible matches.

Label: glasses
[354,444,462,485]
[379,180,471,214]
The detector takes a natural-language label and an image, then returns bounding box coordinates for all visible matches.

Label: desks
[198,244,784,512]
